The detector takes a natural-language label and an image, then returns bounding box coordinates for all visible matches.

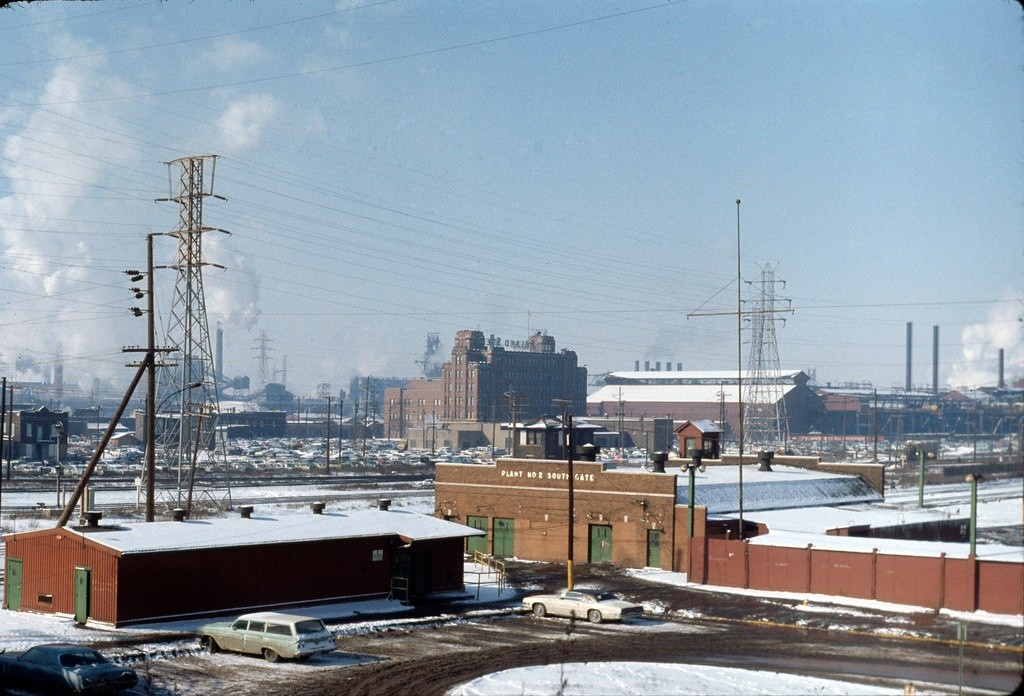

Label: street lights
[145,382,203,522]
[679,463,706,536]
[965,473,986,555]
[915,448,935,507]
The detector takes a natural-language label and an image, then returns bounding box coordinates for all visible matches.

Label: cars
[593,447,649,460]
[196,612,339,665]
[522,588,644,623]
[0,643,139,696]
[722,440,906,459]
[63,436,512,476]
[2,458,52,476]
